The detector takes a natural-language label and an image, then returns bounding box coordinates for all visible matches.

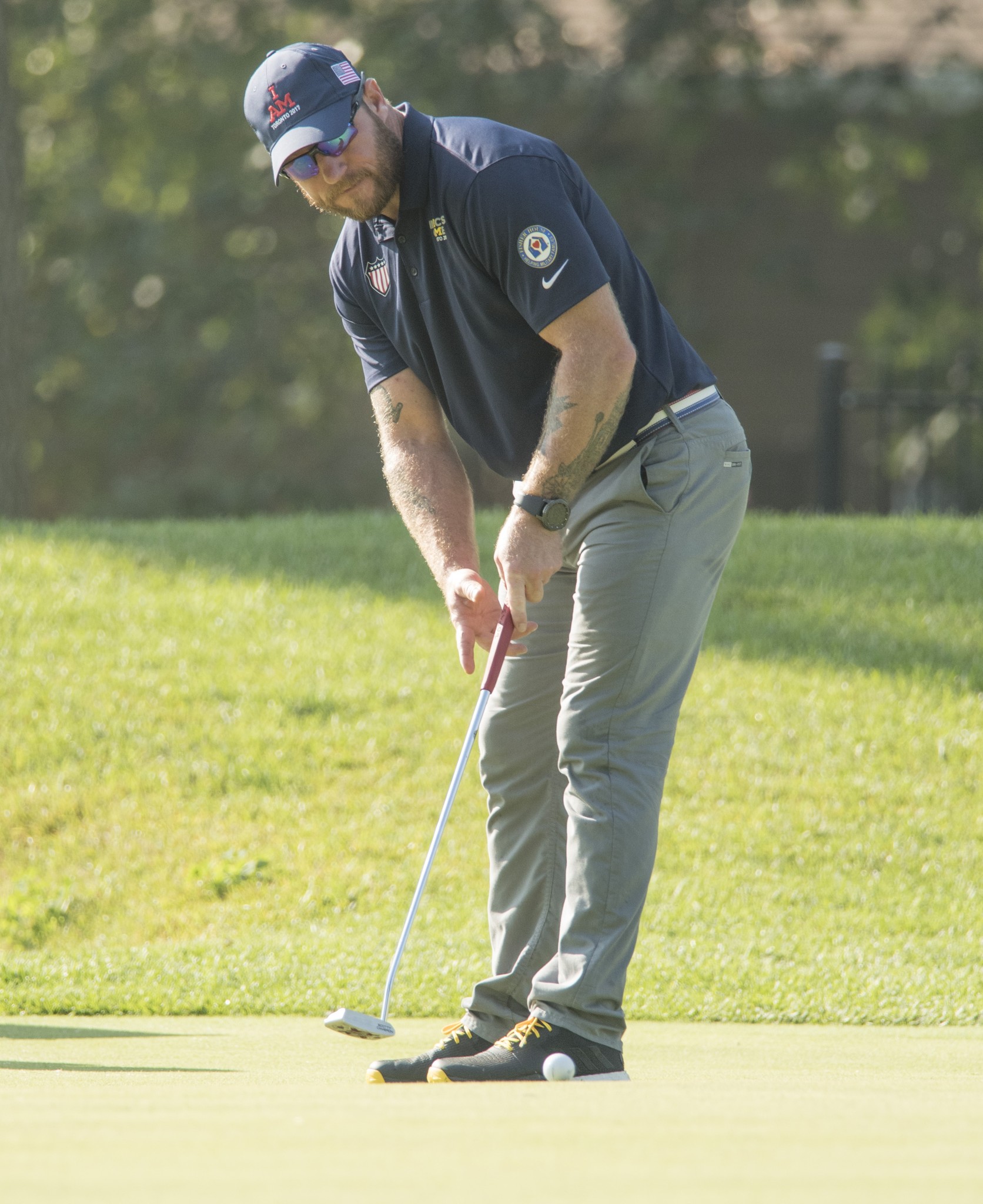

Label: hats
[243,41,367,186]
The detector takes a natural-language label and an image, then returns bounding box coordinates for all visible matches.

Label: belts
[590,384,720,474]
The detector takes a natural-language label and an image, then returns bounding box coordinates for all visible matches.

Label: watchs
[512,492,571,532]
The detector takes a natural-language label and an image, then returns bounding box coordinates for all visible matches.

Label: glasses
[279,100,360,182]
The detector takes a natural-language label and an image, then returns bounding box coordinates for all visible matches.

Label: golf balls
[542,1052,578,1082]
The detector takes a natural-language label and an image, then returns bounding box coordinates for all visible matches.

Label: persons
[244,42,753,1084]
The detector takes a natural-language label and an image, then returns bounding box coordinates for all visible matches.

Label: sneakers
[427,1014,631,1083]
[365,1021,494,1083]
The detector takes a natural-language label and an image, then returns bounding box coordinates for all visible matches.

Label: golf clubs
[324,603,512,1048]
